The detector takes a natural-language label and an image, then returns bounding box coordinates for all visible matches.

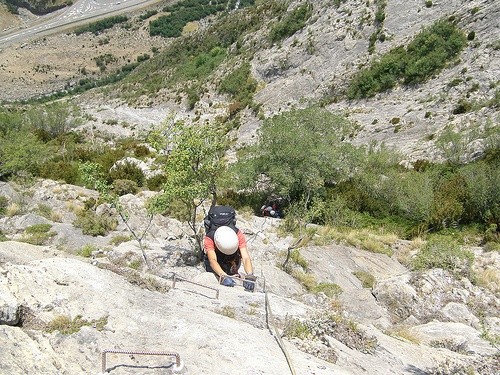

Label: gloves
[243,275,257,290]
[220,276,236,287]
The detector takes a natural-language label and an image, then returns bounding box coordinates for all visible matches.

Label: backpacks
[203,206,239,239]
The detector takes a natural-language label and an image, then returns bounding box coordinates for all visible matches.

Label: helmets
[270,210,275,215]
[214,226,239,255]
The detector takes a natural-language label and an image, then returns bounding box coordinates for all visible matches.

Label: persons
[262,204,281,219]
[203,226,256,290]
